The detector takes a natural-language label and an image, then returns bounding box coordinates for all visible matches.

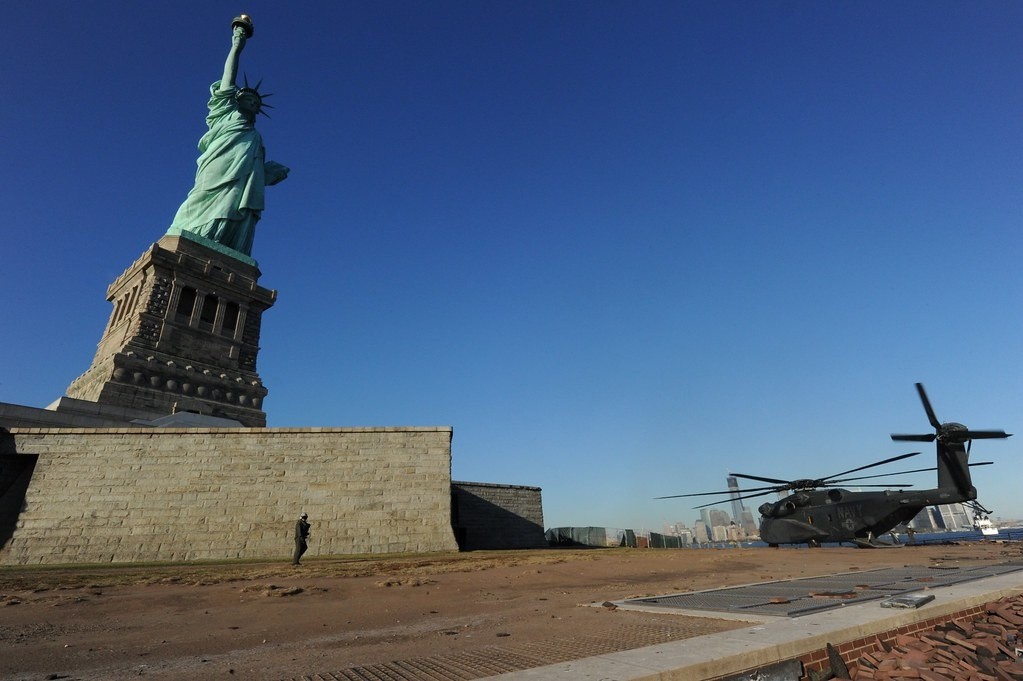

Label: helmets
[300,512,308,521]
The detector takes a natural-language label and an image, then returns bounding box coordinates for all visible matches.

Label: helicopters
[654,381,1016,549]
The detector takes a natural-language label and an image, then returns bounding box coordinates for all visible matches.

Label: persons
[292,512,311,566]
[166,13,290,256]
